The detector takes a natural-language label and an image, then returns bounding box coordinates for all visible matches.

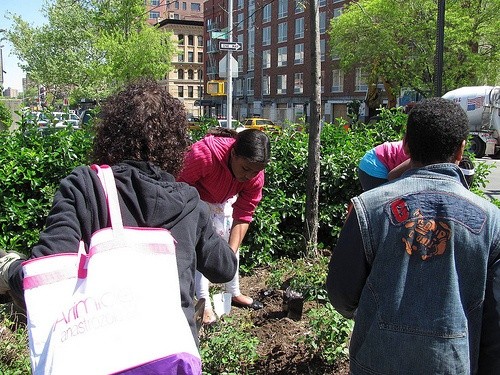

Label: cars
[206,120,244,134]
[27,102,92,132]
[241,116,282,136]
[184,117,204,130]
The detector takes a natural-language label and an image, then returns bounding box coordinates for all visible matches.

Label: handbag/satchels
[21,164,203,375]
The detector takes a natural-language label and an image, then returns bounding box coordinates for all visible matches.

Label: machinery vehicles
[443,84,500,159]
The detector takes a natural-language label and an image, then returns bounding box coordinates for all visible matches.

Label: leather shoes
[231,293,264,310]
[202,310,221,332]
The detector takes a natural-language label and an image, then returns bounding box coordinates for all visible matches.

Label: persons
[176,129,271,332]
[0,79,237,375]
[326,97,500,375]
[358,140,411,192]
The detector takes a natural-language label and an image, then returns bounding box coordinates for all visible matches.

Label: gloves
[0,249,26,294]
